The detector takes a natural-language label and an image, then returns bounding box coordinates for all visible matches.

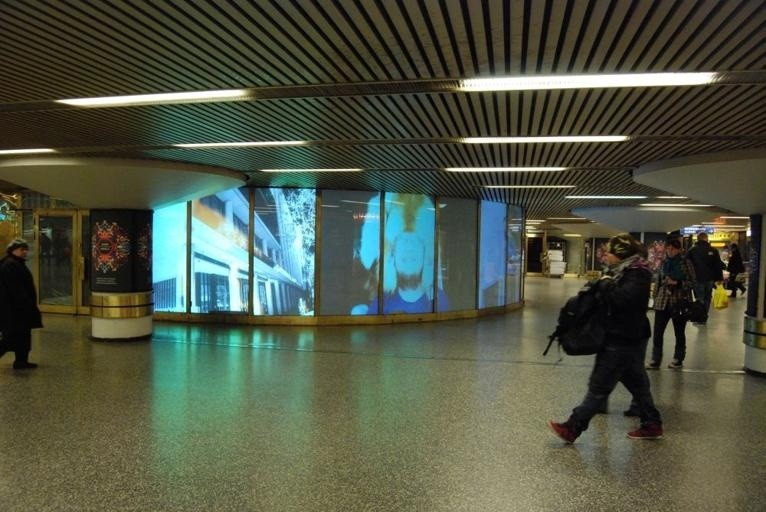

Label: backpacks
[556,277,609,355]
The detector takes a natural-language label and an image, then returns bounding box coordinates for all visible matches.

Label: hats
[606,233,637,259]
[5,238,27,253]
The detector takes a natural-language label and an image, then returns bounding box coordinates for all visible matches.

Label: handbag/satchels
[676,300,703,320]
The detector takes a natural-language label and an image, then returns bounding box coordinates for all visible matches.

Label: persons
[685,232,723,325]
[645,237,696,370]
[357,188,444,291]
[365,229,450,315]
[547,233,663,444]
[0,235,45,371]
[726,244,746,299]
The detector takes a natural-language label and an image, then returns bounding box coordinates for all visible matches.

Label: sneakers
[627,421,664,439]
[549,420,577,444]
[668,358,684,370]
[644,359,659,370]
